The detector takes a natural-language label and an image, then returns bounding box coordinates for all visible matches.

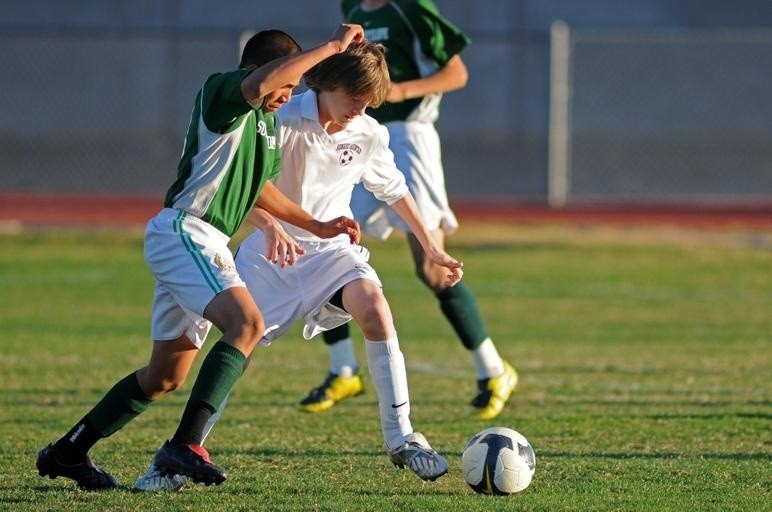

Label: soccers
[463,427,536,496]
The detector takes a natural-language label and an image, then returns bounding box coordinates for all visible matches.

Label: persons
[297,1,520,420]
[37,22,365,491]
[131,38,465,491]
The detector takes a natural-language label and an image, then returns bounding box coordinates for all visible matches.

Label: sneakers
[37,443,117,491]
[384,433,448,480]
[467,362,518,421]
[155,438,226,486]
[131,468,182,495]
[297,369,364,414]
